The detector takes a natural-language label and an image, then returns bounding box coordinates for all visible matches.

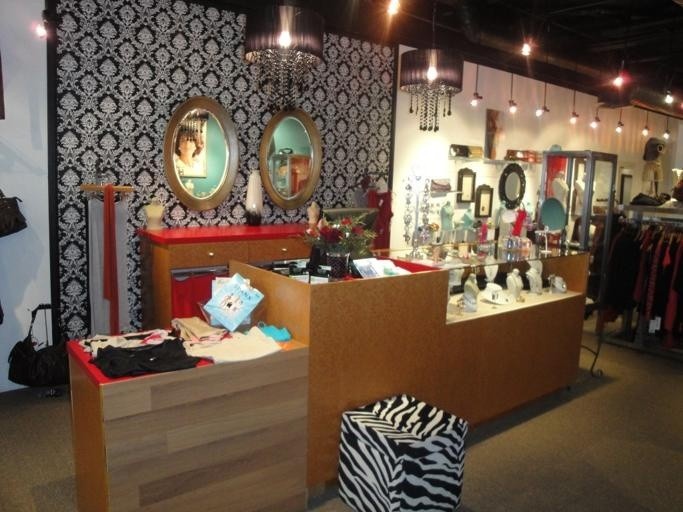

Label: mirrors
[457,168,476,202]
[165,96,239,212]
[498,162,526,210]
[475,184,494,218]
[258,105,326,210]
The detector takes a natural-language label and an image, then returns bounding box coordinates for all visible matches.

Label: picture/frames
[174,118,208,178]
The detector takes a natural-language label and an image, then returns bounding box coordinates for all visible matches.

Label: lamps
[244,4,324,112]
[642,110,651,136]
[663,116,671,139]
[398,1,464,133]
[615,104,625,133]
[569,90,578,124]
[509,73,518,113]
[590,98,603,128]
[470,64,483,106]
[535,81,550,118]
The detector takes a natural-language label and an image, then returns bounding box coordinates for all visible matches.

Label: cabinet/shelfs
[66,322,308,512]
[537,149,618,318]
[228,241,589,489]
[136,220,323,334]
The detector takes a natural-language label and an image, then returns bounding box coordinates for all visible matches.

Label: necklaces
[553,280,566,291]
[510,273,526,303]
[462,294,477,306]
[530,271,543,293]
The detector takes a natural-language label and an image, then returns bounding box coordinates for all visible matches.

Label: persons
[175,125,204,175]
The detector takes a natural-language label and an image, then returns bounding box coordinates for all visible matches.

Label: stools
[336,393,469,511]
